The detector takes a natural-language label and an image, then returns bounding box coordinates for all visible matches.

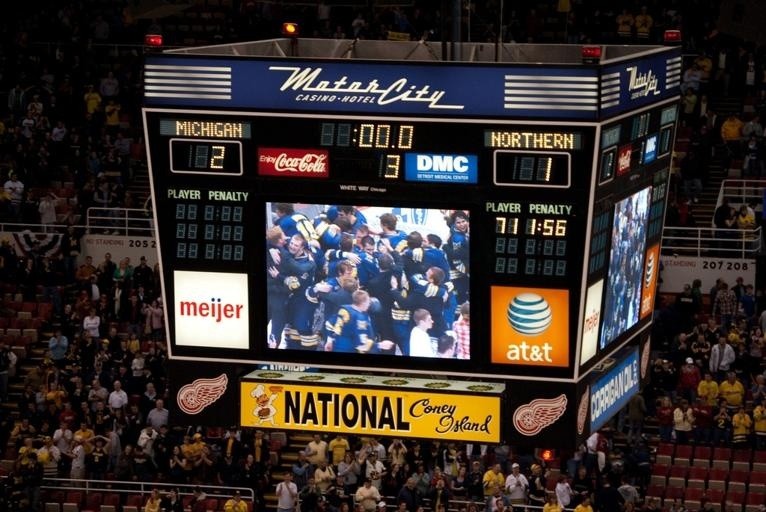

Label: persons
[1,0,766,511]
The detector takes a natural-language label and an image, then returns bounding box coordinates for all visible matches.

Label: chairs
[0,284,65,359]
[44,489,252,512]
[26,181,77,221]
[644,440,766,512]
